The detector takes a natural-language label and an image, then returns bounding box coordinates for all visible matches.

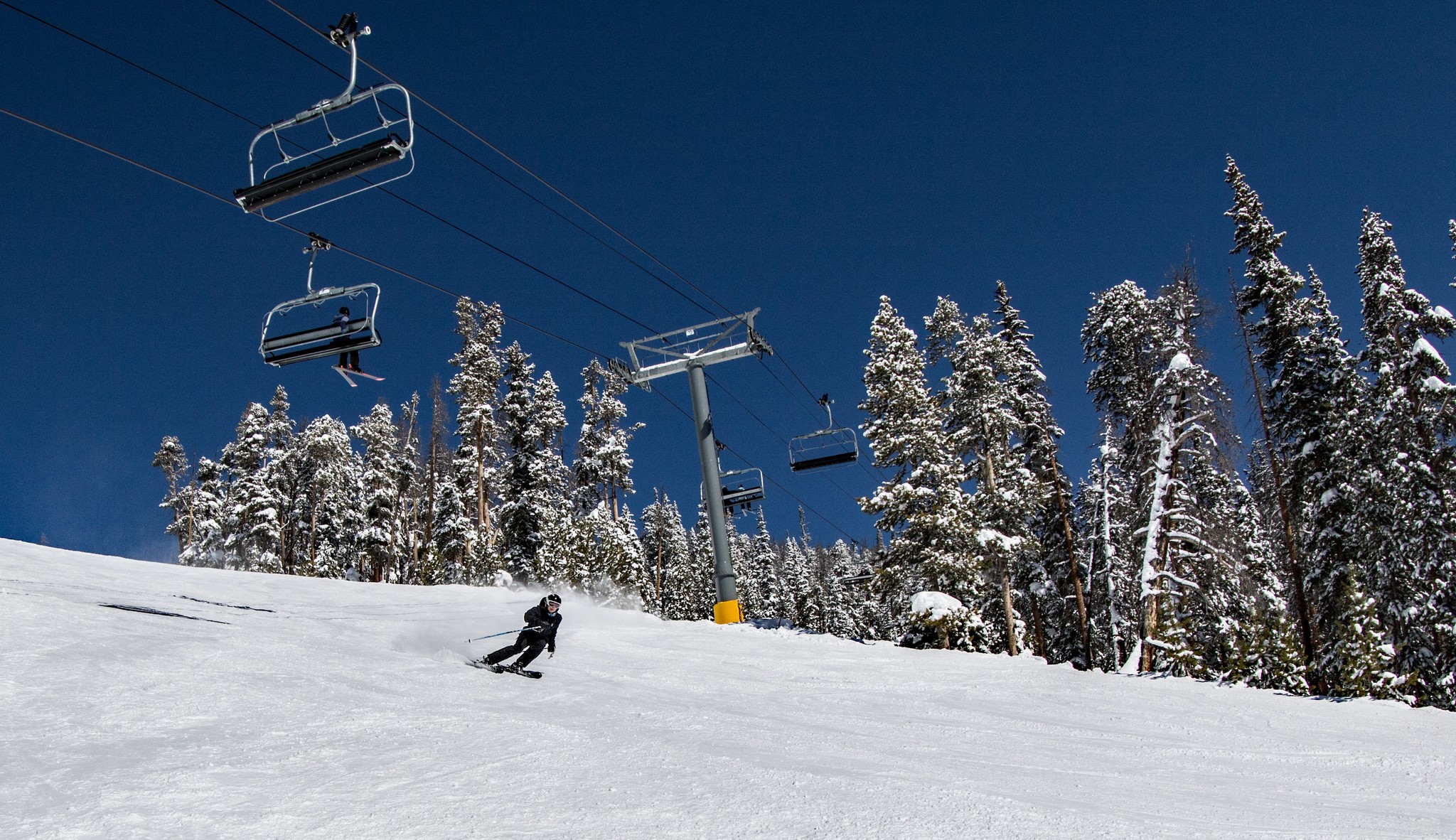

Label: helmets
[548,594,561,605]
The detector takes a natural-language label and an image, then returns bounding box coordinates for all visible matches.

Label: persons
[736,484,752,512]
[475,594,562,674]
[721,485,735,516]
[332,306,364,376]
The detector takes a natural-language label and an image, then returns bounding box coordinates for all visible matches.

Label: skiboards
[463,656,542,679]
[741,508,757,517]
[331,364,386,387]
[724,514,741,519]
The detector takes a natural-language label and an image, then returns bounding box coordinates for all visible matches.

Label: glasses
[547,600,560,609]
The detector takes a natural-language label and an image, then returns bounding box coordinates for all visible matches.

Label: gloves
[538,621,550,630]
[547,645,554,652]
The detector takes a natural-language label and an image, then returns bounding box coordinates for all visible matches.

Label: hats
[339,307,350,316]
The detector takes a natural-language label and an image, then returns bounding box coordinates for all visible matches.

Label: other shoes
[484,657,498,666]
[509,662,523,672]
[349,367,361,373]
[338,364,348,375]
